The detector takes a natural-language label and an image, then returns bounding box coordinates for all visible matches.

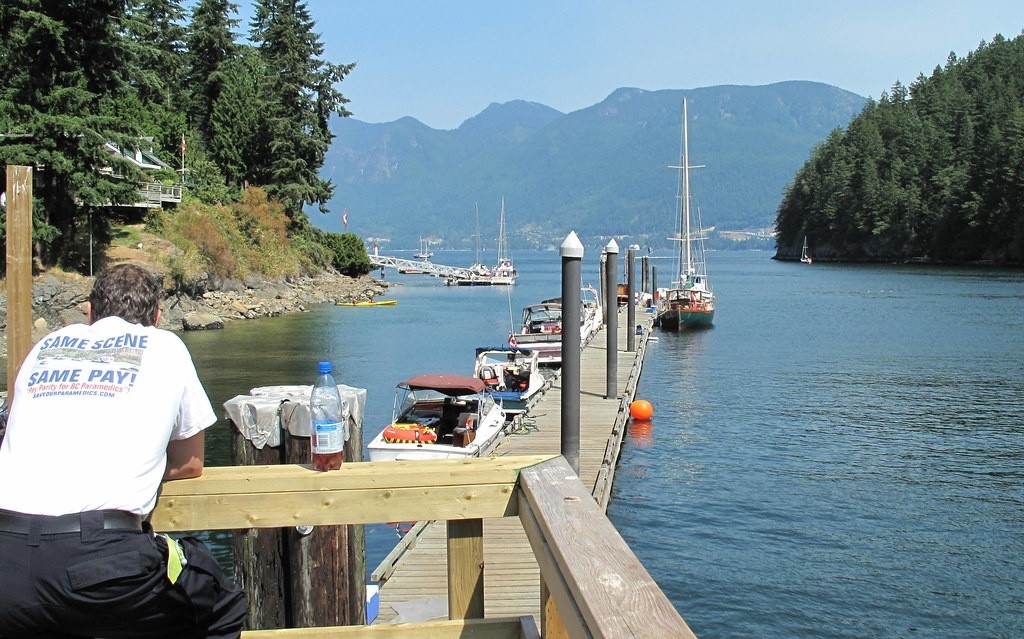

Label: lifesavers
[703,303,708,311]
[383,424,437,445]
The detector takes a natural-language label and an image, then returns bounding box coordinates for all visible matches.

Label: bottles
[308,361,344,471]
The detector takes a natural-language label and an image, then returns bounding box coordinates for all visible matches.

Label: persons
[0,262,251,639]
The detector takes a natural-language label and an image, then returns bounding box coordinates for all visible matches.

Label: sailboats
[801,235,813,264]
[413,236,434,258]
[455,193,520,285]
[656,97,718,330]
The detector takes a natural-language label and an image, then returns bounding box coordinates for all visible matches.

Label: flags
[343,209,348,227]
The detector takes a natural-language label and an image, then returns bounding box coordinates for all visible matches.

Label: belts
[0,511,143,534]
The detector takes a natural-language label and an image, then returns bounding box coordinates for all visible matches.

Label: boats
[365,373,507,459]
[472,344,546,414]
[506,283,605,364]
